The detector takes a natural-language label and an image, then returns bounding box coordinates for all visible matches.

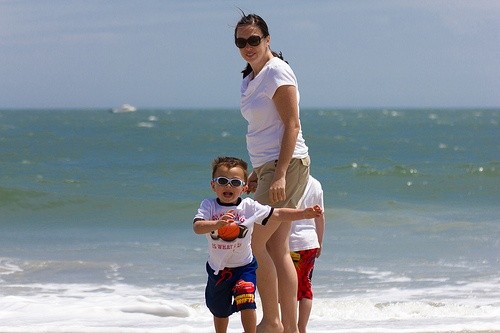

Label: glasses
[235,36,264,48]
[212,176,245,187]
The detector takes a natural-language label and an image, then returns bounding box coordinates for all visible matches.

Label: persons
[193,155,324,333]
[234,7,311,333]
[278,174,325,333]
